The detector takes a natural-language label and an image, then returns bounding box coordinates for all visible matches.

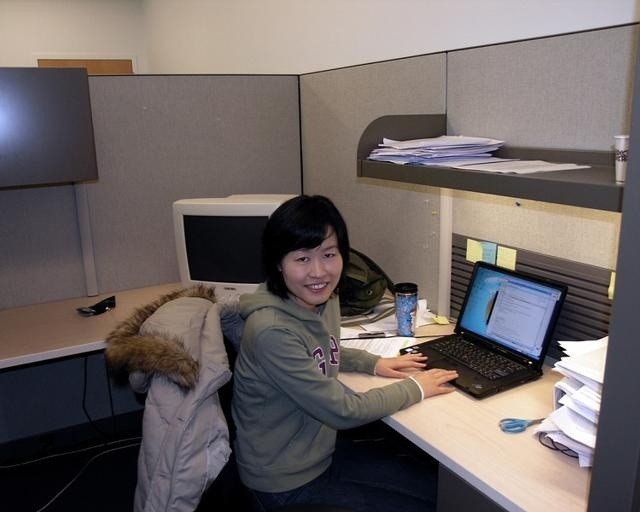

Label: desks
[0,281,591,510]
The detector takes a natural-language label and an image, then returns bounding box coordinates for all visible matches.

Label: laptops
[399,260,569,399]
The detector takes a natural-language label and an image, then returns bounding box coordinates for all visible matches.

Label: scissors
[499,418,546,433]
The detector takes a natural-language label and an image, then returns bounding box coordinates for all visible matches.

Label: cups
[394,282,418,336]
[613,135,629,182]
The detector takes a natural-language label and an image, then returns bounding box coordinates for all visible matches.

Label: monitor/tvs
[172,194,301,299]
[0,65,100,190]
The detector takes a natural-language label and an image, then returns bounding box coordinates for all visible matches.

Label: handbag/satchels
[333,246,396,317]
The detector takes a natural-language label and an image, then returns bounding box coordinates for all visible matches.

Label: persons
[221,192,460,512]
